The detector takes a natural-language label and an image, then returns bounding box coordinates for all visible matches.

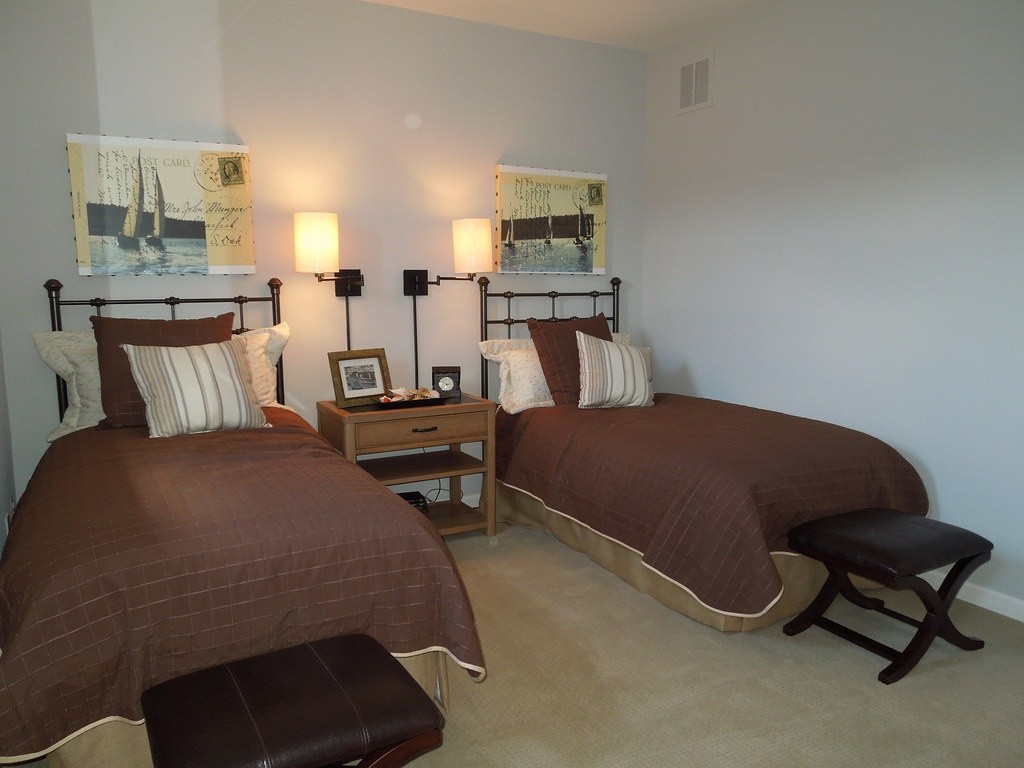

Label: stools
[783,507,994,682]
[141,633,446,768]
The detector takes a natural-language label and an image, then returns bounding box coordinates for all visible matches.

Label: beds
[0,278,487,765]
[477,277,931,634]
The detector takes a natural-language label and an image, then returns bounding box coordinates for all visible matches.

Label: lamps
[403,218,493,389]
[293,212,365,350]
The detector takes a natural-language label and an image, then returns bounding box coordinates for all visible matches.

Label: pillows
[32,322,291,443]
[119,334,274,439]
[89,312,235,430]
[576,331,655,409]
[477,332,632,415]
[525,312,613,407]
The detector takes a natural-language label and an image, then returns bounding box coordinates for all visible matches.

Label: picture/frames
[327,347,394,409]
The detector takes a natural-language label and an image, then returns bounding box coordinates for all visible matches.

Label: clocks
[432,366,461,399]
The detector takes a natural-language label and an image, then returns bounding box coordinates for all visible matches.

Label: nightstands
[316,392,497,537]
